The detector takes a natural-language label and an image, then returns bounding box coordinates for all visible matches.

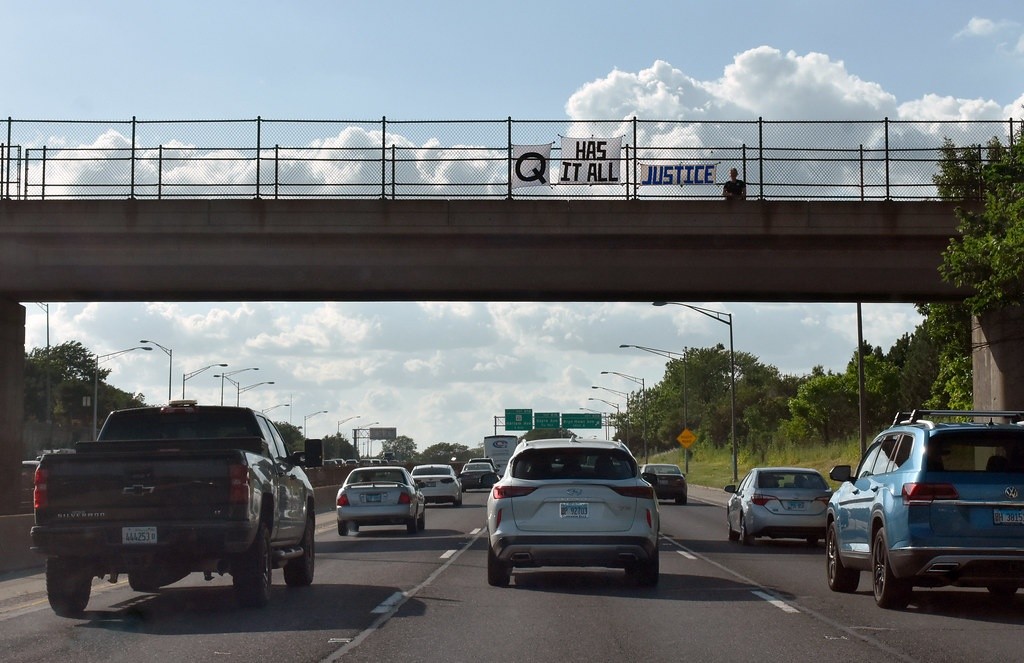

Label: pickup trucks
[29,400,317,617]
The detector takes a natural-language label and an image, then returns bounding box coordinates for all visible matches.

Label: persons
[723,168,746,200]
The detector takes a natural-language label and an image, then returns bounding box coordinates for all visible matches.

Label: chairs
[529,457,625,479]
[648,470,655,474]
[800,479,817,489]
[985,456,1009,473]
[761,475,779,488]
[926,457,944,472]
[667,470,675,474]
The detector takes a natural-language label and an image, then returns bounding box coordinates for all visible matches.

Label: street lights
[578,300,738,483]
[93,335,377,458]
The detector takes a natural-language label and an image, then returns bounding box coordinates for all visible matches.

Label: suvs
[468,457,499,474]
[823,408,1024,611]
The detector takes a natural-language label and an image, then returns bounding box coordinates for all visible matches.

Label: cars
[640,463,688,505]
[410,464,464,507]
[723,467,833,547]
[336,466,426,536]
[485,436,661,586]
[458,463,501,492]
[291,457,407,464]
